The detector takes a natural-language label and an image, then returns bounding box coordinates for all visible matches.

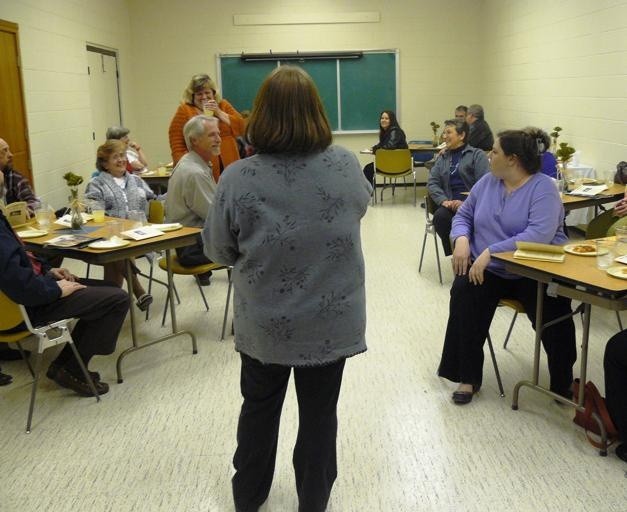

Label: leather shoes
[0,371,13,387]
[451,385,475,405]
[1,348,32,360]
[46,360,109,397]
[554,391,574,405]
[196,278,211,286]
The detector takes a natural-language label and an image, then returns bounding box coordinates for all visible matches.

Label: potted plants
[553,142,578,195]
[431,121,440,147]
[64,172,85,230]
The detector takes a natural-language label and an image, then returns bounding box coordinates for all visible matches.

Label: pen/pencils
[79,238,106,249]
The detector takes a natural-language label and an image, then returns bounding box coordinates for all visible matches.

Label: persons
[199,62,377,512]
[454,105,469,132]
[168,73,248,287]
[434,128,578,404]
[612,185,627,225]
[237,108,258,159]
[1,170,130,398]
[1,136,43,219]
[426,117,493,258]
[420,103,495,210]
[102,122,147,176]
[84,139,171,312]
[164,114,222,269]
[363,110,409,196]
[0,342,31,386]
[602,328,627,462]
[521,125,562,179]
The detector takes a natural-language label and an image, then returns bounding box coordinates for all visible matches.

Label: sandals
[136,294,153,312]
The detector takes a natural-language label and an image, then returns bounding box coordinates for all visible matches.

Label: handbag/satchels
[615,162,627,187]
[573,378,619,448]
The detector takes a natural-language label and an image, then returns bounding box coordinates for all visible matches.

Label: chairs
[448,232,528,397]
[157,252,236,340]
[418,189,448,286]
[0,288,102,432]
[84,200,180,322]
[372,149,419,206]
[409,140,436,188]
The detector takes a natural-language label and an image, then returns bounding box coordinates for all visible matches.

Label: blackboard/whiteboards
[215,48,399,134]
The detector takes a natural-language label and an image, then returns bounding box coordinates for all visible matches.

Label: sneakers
[367,196,376,206]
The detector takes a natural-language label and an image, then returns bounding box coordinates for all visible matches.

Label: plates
[570,178,597,184]
[564,243,608,256]
[88,241,129,249]
[71,212,94,220]
[607,266,626,279]
[155,224,184,231]
[17,230,46,238]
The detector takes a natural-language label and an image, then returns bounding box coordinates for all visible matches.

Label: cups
[606,174,614,188]
[203,100,214,116]
[108,220,123,240]
[93,201,105,223]
[595,239,617,270]
[557,179,564,196]
[130,209,143,228]
[158,162,166,176]
[36,209,49,228]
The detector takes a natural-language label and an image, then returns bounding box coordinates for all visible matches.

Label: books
[566,184,609,198]
[513,239,568,264]
[119,226,165,242]
[55,214,73,228]
[45,233,102,247]
